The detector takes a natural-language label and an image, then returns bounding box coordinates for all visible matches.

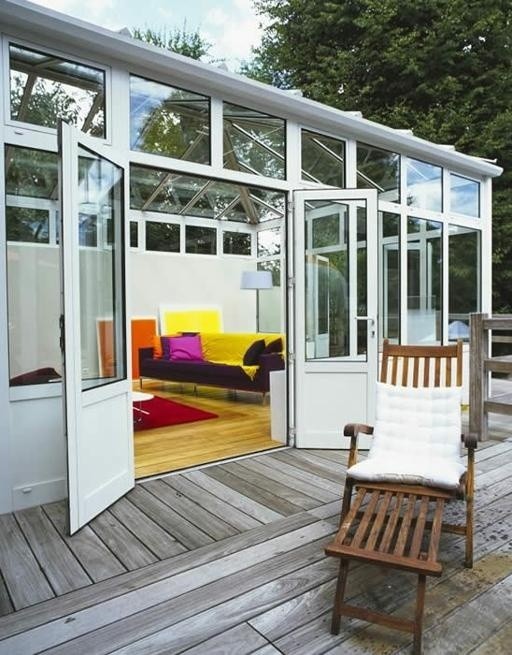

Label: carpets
[134,394,219,432]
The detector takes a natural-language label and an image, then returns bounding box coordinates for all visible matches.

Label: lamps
[239,270,273,332]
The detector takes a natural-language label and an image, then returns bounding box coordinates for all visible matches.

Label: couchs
[138,331,286,405]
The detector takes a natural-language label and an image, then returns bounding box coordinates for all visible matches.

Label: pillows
[150,335,204,362]
[347,385,469,494]
[242,336,284,366]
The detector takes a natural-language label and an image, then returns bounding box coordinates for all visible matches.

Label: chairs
[338,338,484,570]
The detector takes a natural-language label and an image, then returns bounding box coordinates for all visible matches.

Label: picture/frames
[95,305,224,383]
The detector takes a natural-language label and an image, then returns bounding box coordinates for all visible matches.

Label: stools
[324,485,450,655]
